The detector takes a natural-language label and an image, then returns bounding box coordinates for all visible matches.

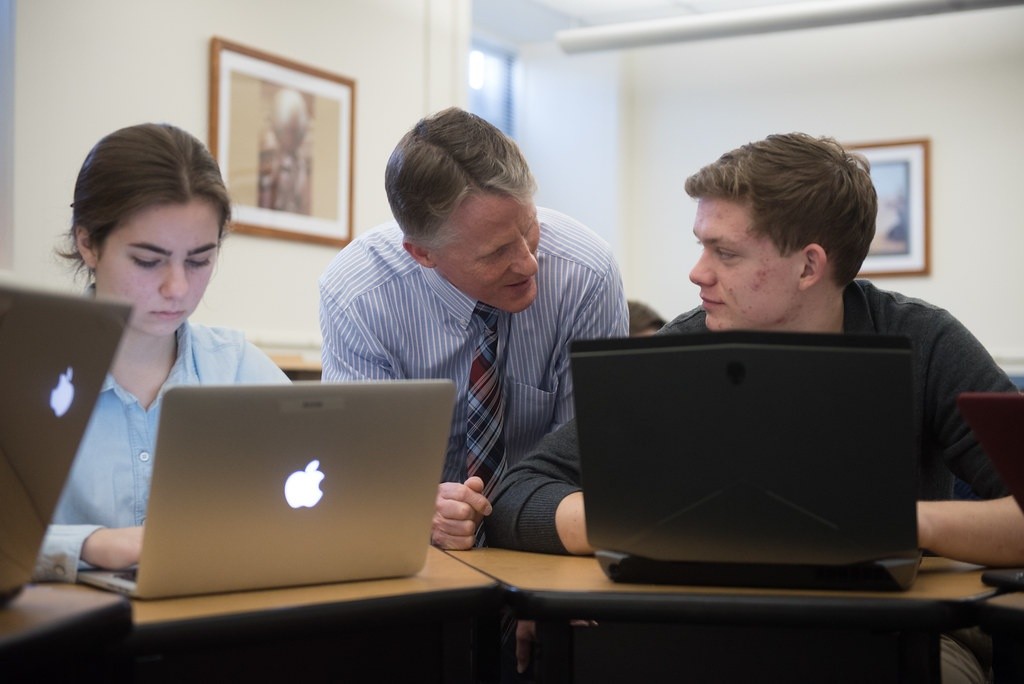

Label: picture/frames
[840,137,934,277]
[206,36,359,246]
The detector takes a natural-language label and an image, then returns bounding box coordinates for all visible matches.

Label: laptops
[79,379,455,598]
[0,275,135,607]
[569,330,925,592]
[961,391,1024,516]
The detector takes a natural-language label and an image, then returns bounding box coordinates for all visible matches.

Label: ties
[465,300,508,548]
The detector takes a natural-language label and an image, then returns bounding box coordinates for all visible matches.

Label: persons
[627,299,669,338]
[322,104,630,684]
[26,124,299,582]
[486,133,1024,683]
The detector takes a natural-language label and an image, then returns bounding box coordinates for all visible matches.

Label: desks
[446,546,1004,683]
[129,540,498,684]
